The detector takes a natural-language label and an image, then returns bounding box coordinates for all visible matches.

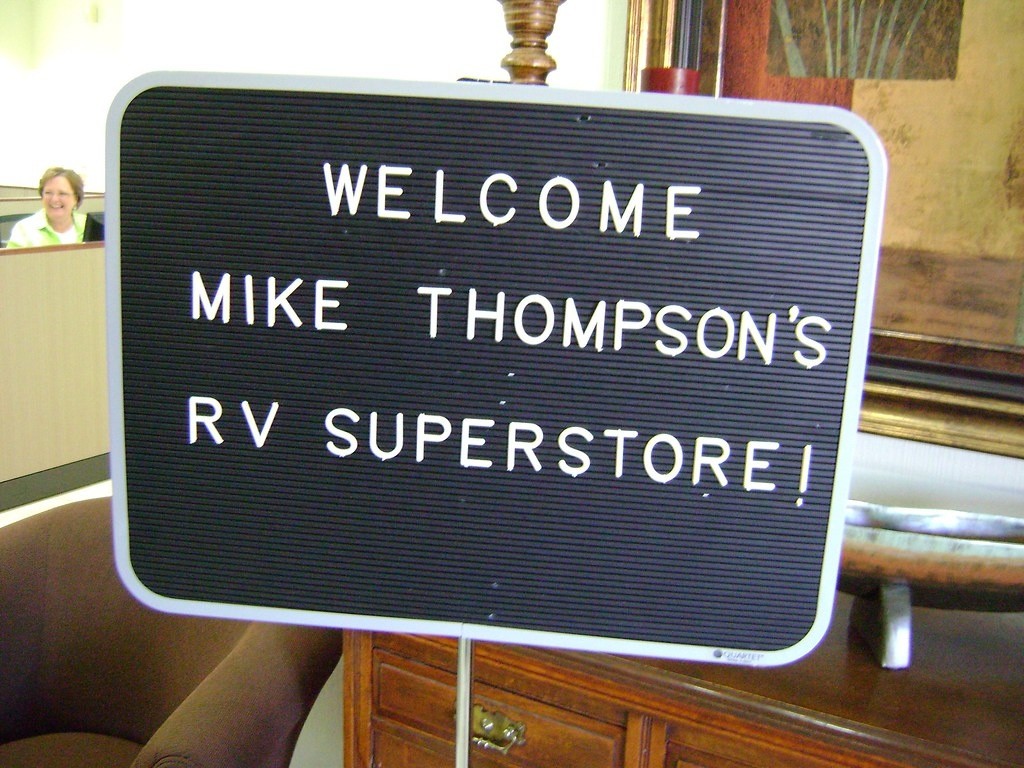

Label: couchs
[0,496,342,768]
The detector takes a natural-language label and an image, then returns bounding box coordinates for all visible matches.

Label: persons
[6,166,87,249]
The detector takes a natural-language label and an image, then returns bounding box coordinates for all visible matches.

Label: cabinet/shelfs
[342,591,1023,768]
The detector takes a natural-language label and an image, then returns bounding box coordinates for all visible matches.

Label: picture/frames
[623,0,1024,459]
